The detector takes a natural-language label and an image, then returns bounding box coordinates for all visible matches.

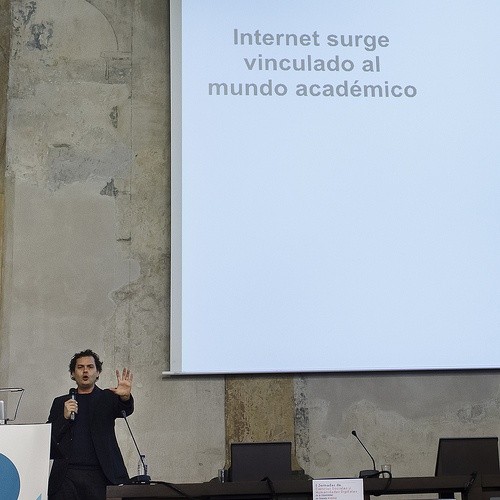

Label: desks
[106,480,312,500]
[363,474,500,500]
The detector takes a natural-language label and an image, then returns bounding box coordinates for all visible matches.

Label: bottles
[138,455,148,483]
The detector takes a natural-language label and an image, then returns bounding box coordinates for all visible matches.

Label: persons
[46,349,135,500]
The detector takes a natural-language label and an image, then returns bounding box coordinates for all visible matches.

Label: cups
[218,469,228,484]
[382,464,391,478]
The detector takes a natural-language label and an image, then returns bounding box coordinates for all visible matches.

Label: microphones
[69,388,76,422]
[352,430,375,470]
[120,409,147,475]
